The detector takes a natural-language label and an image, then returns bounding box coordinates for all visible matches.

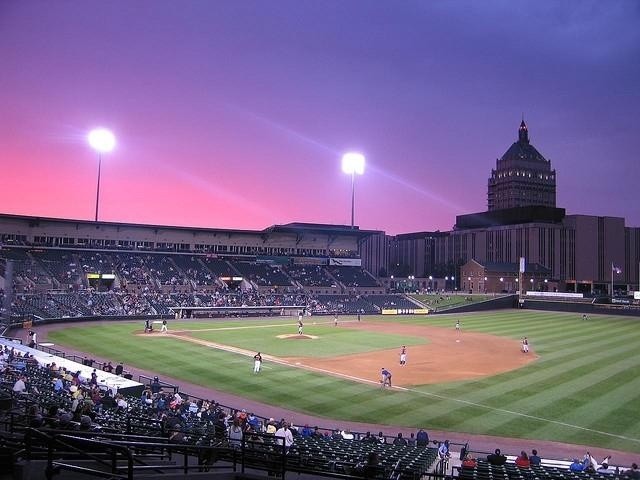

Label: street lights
[411,275,414,289]
[445,276,448,293]
[516,278,518,291]
[390,275,396,291]
[429,276,432,293]
[530,279,534,290]
[342,152,365,229]
[544,279,547,292]
[408,275,411,290]
[451,276,454,293]
[499,277,503,294]
[468,276,471,292]
[485,277,488,293]
[87,127,115,223]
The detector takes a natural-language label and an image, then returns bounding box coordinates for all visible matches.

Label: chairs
[1,331,90,479]
[292,428,468,479]
[1,236,47,330]
[196,399,293,479]
[47,243,428,316]
[87,362,195,479]
[458,453,640,479]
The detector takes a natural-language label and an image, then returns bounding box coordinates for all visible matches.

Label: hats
[174,423,182,428]
